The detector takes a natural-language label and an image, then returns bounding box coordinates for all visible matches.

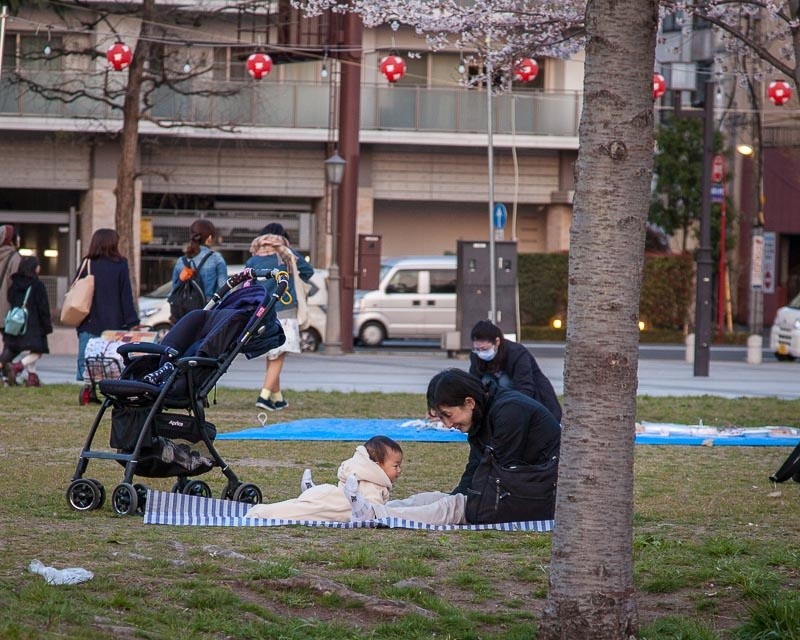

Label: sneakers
[255,396,277,412]
[276,400,289,410]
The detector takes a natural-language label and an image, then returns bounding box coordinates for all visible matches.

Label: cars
[137,263,328,353]
[770,292,800,362]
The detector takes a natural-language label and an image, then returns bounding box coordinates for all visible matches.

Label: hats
[0,225,14,245]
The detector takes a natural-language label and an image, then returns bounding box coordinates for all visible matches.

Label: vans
[346,254,463,349]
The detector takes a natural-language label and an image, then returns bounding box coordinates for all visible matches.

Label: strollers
[63,267,293,516]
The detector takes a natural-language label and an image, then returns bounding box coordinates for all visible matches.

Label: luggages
[78,320,160,405]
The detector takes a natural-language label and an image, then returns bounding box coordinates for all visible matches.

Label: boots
[6,360,23,385]
[27,372,39,386]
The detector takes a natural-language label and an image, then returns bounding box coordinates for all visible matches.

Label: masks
[472,342,498,360]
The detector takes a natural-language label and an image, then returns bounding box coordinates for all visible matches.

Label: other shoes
[89,397,103,404]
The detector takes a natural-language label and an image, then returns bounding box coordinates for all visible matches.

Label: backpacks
[167,251,215,325]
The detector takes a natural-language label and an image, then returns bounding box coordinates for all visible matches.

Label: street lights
[321,150,349,347]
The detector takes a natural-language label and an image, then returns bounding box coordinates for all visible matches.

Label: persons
[246,223,315,412]
[301,369,563,526]
[60,229,141,404]
[249,436,404,522]
[6,257,53,389]
[469,320,562,425]
[0,225,23,393]
[168,219,228,328]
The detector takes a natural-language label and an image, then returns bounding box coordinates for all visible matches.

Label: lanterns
[107,42,133,70]
[653,72,667,100]
[767,79,794,105]
[511,58,539,82]
[246,53,273,79]
[381,55,407,83]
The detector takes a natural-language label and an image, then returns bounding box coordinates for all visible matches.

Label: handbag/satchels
[286,248,312,325]
[60,258,95,326]
[476,446,558,524]
[4,308,28,335]
